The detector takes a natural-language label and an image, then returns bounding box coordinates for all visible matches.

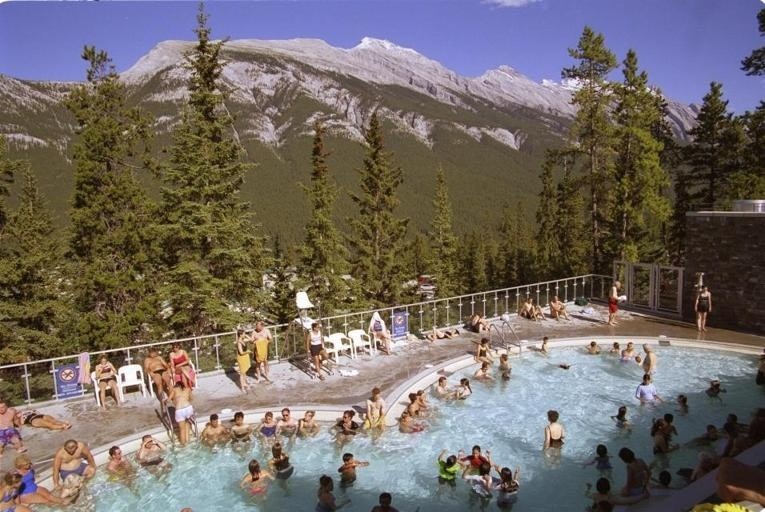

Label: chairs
[145,366,173,401]
[348,330,373,361]
[327,333,354,364]
[295,291,317,326]
[117,364,148,402]
[318,337,339,369]
[367,328,391,356]
[89,369,122,407]
[168,358,197,388]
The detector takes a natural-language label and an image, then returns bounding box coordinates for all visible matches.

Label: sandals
[319,358,325,381]
